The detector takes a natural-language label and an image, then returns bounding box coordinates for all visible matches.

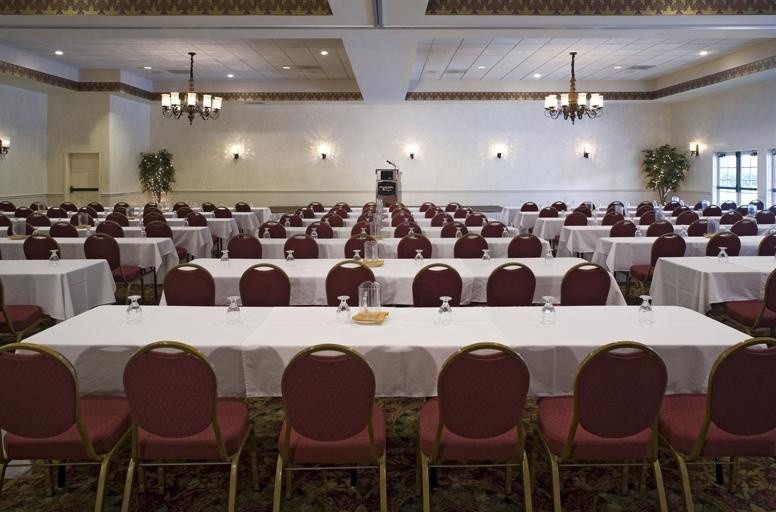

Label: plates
[352,314,378,324]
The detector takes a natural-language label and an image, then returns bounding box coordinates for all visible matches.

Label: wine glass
[334,295,351,325]
[125,294,143,325]
[437,297,455,324]
[224,296,242,324]
[540,296,556,326]
[8,197,776,268]
[637,293,654,324]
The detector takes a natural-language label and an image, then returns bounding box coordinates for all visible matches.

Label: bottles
[358,281,382,320]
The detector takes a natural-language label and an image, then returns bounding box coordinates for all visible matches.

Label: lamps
[689,142,699,157]
[544,52,604,124]
[231,145,238,159]
[497,145,503,158]
[584,144,589,158]
[409,144,416,159]
[160,51,223,127]
[320,146,327,159]
[0,136,10,154]
